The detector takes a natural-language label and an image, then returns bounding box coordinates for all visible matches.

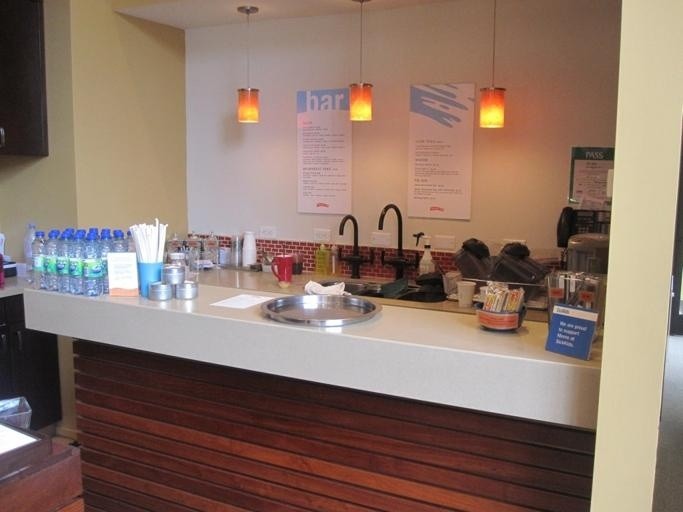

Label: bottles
[24,221,257,302]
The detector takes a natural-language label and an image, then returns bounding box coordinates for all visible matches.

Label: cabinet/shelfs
[1,293,62,431]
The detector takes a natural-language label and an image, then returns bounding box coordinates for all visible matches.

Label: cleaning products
[412,230,436,278]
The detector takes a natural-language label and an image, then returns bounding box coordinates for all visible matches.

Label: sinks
[355,284,414,299]
[319,279,365,295]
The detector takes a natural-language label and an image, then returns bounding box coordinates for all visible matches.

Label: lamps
[478,1,507,129]
[236,5,261,124]
[348,0,374,123]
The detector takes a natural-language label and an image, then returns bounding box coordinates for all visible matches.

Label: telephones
[555,207,609,248]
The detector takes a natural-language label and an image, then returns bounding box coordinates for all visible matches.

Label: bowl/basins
[475,302,527,332]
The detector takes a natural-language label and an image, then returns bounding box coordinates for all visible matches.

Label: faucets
[378,203,420,284]
[337,214,375,278]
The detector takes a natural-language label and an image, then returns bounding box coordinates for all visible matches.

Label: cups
[271,255,294,285]
[456,281,476,308]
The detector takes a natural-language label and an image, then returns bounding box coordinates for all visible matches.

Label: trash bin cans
[0,396,34,430]
